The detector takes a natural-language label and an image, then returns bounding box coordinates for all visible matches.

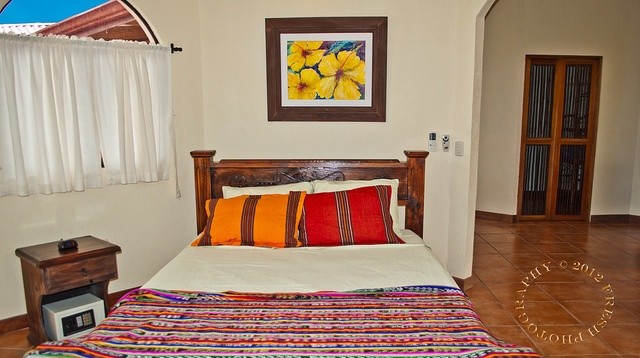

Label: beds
[23,149,543,358]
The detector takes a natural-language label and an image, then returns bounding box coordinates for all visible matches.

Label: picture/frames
[264,16,386,123]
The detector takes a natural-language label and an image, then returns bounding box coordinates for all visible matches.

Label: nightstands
[14,235,121,346]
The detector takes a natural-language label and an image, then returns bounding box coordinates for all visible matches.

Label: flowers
[287,40,366,99]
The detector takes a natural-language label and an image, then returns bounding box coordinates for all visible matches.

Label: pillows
[222,183,312,233]
[313,177,401,236]
[296,185,405,247]
[191,190,305,248]
